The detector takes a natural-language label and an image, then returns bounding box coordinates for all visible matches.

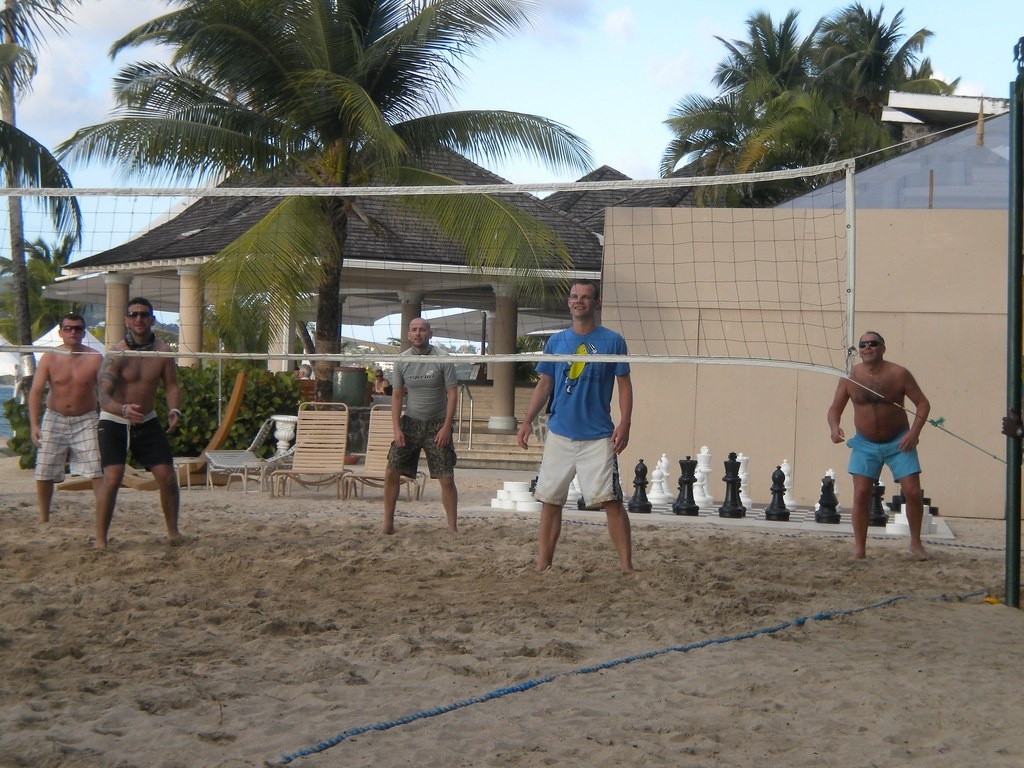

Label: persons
[29,314,104,525]
[92,295,183,549]
[514,281,633,569]
[293,364,312,380]
[382,319,459,533]
[826,331,935,561]
[370,370,393,396]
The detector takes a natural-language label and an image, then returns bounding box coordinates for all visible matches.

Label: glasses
[859,340,882,348]
[61,324,85,332]
[376,375,382,377]
[127,312,150,318]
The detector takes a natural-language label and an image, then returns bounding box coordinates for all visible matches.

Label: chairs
[172,418,273,494]
[204,437,302,497]
[270,401,358,500]
[341,403,429,500]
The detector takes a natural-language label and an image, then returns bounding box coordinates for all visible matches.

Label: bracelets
[123,404,128,418]
[171,408,181,417]
